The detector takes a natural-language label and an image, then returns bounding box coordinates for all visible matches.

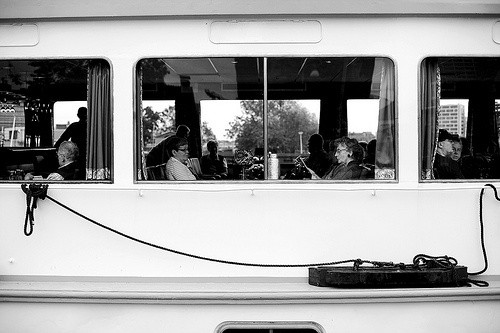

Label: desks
[8,148,57,152]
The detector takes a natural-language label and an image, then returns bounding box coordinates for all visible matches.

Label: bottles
[268,154,279,179]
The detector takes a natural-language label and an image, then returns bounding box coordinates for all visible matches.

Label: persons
[46,141,84,180]
[322,138,365,180]
[0,107,500,179]
[166,137,196,181]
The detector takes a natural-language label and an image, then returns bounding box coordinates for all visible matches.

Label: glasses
[178,148,188,151]
[336,149,348,152]
[56,154,65,158]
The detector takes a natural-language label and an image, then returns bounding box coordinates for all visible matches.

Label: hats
[438,129,458,142]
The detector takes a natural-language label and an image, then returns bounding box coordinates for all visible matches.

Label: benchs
[147,158,203,180]
